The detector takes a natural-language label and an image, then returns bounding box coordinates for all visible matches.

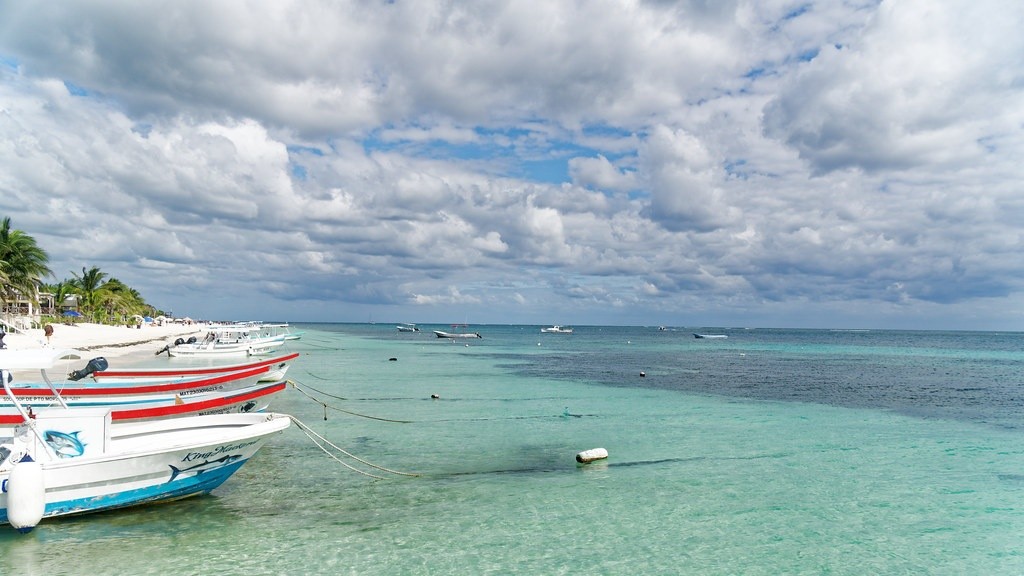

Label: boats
[433,330,483,339]
[0,310,306,537]
[540,325,573,333]
[396,323,420,333]
[692,332,730,340]
